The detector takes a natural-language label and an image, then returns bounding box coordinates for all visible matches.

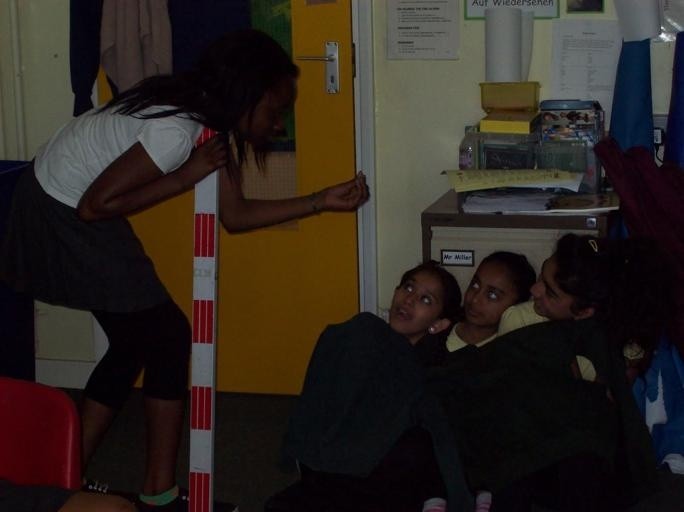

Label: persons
[385,260,462,348]
[413,249,538,510]
[0,26,371,510]
[496,231,654,406]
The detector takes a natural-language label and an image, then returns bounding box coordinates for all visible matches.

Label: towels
[101,0,173,95]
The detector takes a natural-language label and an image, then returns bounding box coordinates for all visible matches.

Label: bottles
[460,126,474,170]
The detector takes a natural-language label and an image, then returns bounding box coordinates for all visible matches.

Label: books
[502,189,619,214]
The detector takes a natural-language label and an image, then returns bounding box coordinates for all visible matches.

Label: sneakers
[80,477,138,503]
[139,485,240,512]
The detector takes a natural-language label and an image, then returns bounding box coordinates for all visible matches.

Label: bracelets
[311,191,321,216]
